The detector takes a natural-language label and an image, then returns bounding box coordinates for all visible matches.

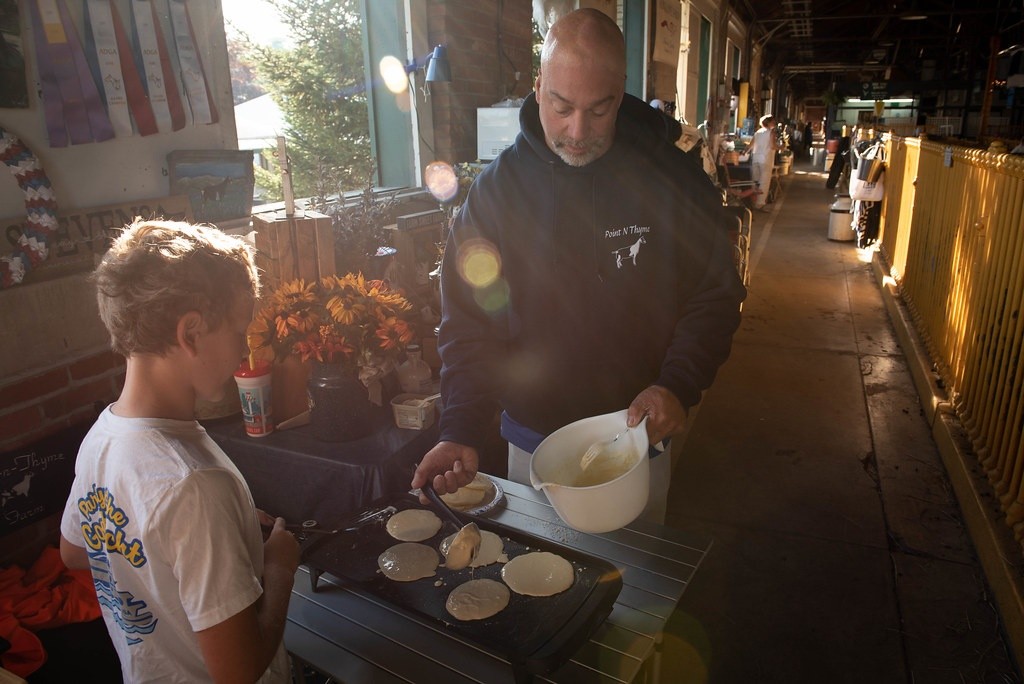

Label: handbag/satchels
[857,147,881,182]
[848,143,885,202]
[867,147,886,185]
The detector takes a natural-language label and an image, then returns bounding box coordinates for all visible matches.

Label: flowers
[439,158,482,219]
[246,270,418,363]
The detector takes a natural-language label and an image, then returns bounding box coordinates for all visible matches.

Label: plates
[410,471,505,515]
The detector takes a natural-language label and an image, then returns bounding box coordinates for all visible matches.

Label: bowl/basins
[390,392,436,431]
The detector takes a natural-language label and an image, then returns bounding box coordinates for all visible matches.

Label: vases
[305,352,371,443]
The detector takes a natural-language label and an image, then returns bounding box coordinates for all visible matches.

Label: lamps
[406,42,453,82]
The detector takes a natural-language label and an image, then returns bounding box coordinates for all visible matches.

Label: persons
[804,121,813,150]
[412,7,747,527]
[742,114,784,213]
[57,216,303,684]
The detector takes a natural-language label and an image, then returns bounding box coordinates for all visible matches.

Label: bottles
[828,194,856,242]
[398,344,434,395]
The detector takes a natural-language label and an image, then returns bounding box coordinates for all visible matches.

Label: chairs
[723,157,763,222]
[722,205,751,313]
[772,166,788,200]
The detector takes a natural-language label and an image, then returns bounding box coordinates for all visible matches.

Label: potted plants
[818,88,848,120]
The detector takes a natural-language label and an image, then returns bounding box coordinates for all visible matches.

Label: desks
[203,390,508,530]
[267,471,713,684]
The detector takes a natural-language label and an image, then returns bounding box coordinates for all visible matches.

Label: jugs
[529,406,665,533]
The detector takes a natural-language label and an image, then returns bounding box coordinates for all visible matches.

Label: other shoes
[753,204,770,213]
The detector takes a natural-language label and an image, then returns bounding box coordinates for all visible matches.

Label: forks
[579,425,634,471]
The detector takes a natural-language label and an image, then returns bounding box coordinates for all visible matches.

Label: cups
[232,359,275,437]
